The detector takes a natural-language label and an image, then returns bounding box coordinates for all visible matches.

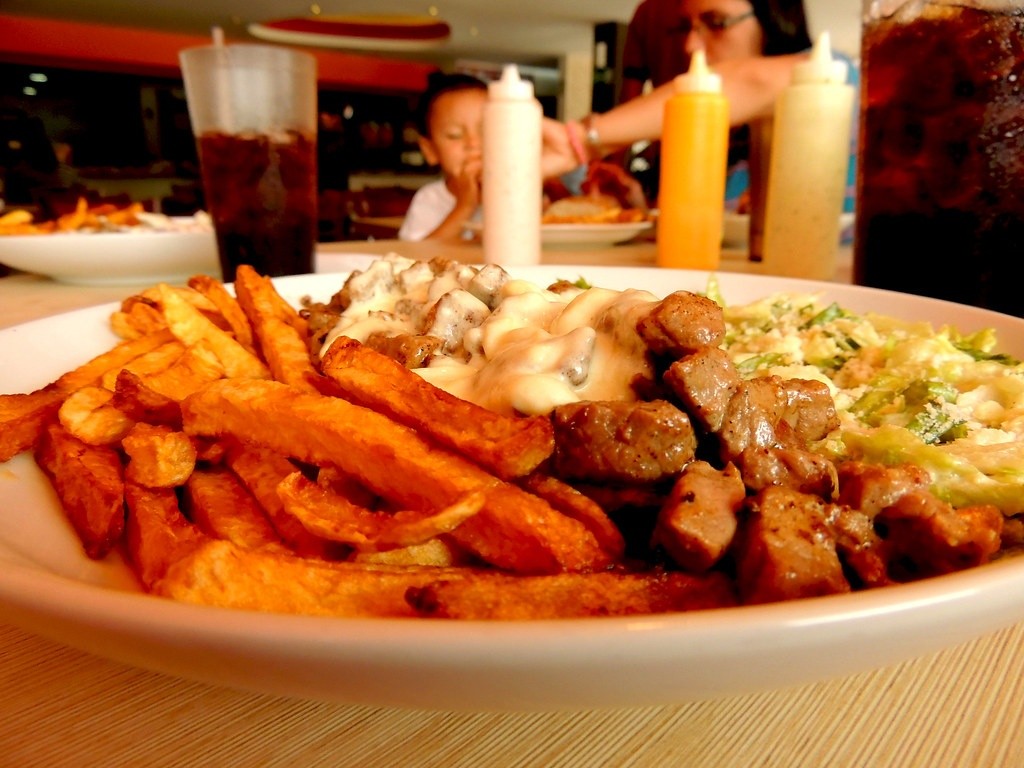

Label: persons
[606,0,691,172]
[398,74,488,241]
[321,107,427,194]
[544,1,832,221]
[0,106,60,202]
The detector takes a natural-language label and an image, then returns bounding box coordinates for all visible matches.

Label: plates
[0,217,221,287]
[649,207,856,246]
[0,264,1024,712]
[466,222,652,248]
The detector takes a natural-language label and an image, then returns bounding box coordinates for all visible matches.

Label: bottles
[656,46,729,252]
[759,30,854,281]
[480,61,543,266]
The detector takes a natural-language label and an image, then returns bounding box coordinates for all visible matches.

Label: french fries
[544,207,658,224]
[0,198,146,237]
[0,264,740,627]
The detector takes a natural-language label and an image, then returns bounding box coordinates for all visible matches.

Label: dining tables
[0,171,1024,768]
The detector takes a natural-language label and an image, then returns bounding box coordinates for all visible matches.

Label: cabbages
[697,280,1024,525]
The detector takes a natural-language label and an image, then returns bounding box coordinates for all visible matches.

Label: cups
[749,115,774,260]
[177,42,318,284]
[853,0,1024,319]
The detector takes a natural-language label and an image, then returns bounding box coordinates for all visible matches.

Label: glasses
[667,10,754,34]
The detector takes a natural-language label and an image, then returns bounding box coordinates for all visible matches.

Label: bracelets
[581,116,602,160]
[566,123,586,164]
